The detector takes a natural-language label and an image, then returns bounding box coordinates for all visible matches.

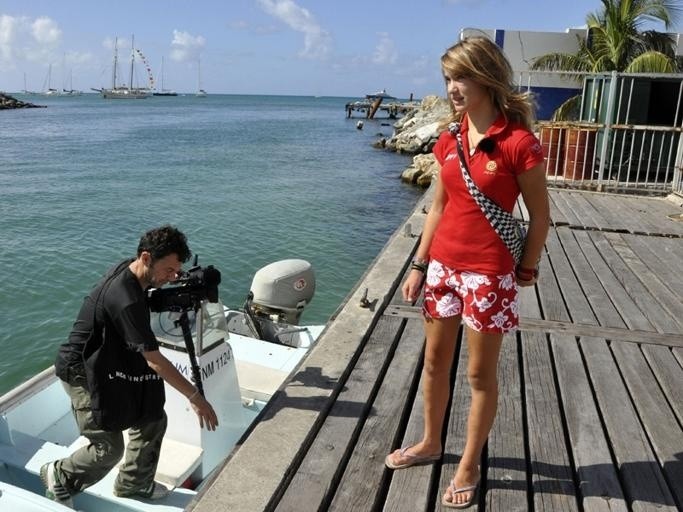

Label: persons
[40,224,218,509]
[385,37,550,509]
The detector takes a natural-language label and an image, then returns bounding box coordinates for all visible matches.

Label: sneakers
[39,461,74,510]
[112,480,169,501]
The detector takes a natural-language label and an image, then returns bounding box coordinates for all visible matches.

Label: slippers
[442,470,482,509]
[385,446,441,470]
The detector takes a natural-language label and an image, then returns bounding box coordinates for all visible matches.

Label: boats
[0,259,325,512]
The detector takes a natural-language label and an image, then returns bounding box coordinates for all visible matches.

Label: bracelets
[409,256,428,273]
[516,267,535,281]
[188,385,199,401]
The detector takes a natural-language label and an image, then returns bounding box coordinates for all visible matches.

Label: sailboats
[21,34,211,99]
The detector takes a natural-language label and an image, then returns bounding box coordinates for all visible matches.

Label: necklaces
[468,132,476,156]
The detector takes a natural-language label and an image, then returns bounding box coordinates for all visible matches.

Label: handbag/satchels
[83,330,167,431]
[449,123,529,268]
